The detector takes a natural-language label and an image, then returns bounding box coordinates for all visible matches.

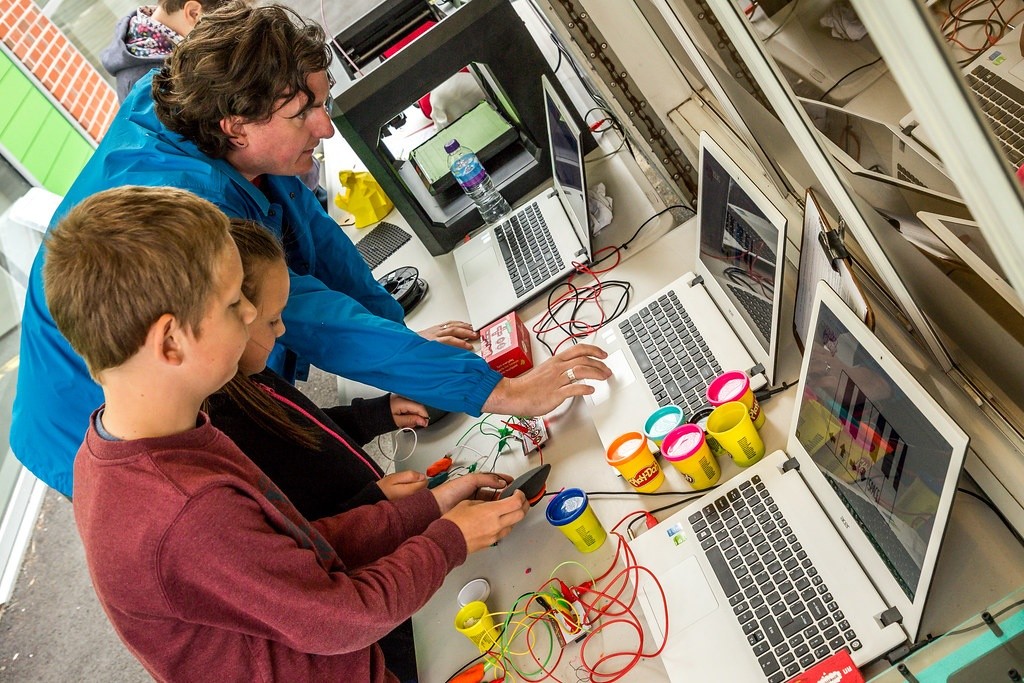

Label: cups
[796,387,941,530]
[545,488,606,554]
[454,601,501,652]
[605,432,665,495]
[644,370,765,490]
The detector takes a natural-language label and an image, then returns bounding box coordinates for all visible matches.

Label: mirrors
[729,0,1024,344]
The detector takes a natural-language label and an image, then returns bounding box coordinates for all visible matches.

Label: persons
[9,0,612,503]
[40,184,530,683]
[200,218,429,683]
[808,341,903,406]
[99,0,232,108]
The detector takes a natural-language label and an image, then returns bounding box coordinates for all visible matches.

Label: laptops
[451,74,593,333]
[569,130,789,478]
[617,278,970,683]
[794,17,1024,320]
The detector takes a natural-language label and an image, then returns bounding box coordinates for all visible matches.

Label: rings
[566,369,578,384]
[825,361,830,372]
[444,325,447,329]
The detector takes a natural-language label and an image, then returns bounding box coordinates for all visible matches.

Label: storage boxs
[479,312,534,378]
[784,648,866,683]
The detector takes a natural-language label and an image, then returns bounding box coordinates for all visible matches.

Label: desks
[730,0,1024,201]
[321,0,1024,683]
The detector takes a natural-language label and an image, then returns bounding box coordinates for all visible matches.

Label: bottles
[445,139,512,227]
